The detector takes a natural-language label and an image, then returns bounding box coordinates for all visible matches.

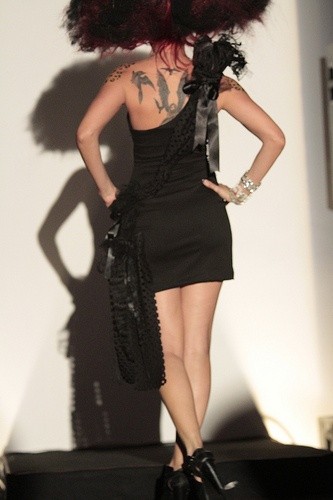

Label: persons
[62,0,286,500]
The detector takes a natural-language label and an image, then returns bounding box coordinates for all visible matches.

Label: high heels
[187,449,224,492]
[156,465,203,495]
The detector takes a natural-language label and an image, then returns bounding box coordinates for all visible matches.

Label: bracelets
[229,185,248,205]
[239,173,261,192]
